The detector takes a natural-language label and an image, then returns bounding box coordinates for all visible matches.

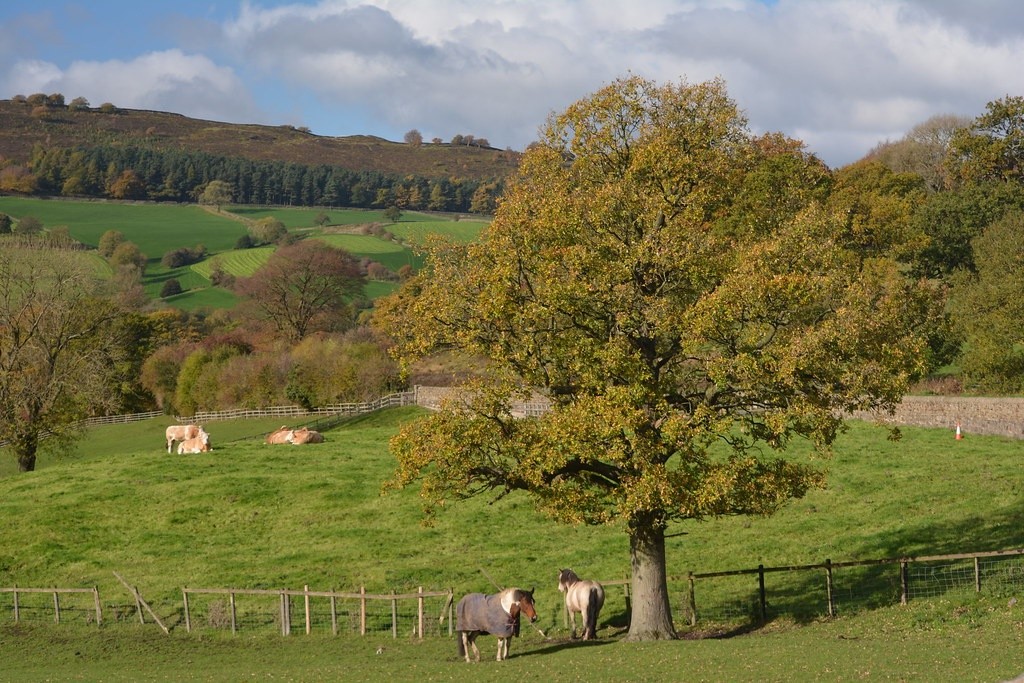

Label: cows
[165,419,214,454]
[265,426,324,444]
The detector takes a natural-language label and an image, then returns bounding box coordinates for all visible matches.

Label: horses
[558,568,605,639]
[456,587,537,661]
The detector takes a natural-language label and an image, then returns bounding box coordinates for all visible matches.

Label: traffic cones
[955,421,964,439]
